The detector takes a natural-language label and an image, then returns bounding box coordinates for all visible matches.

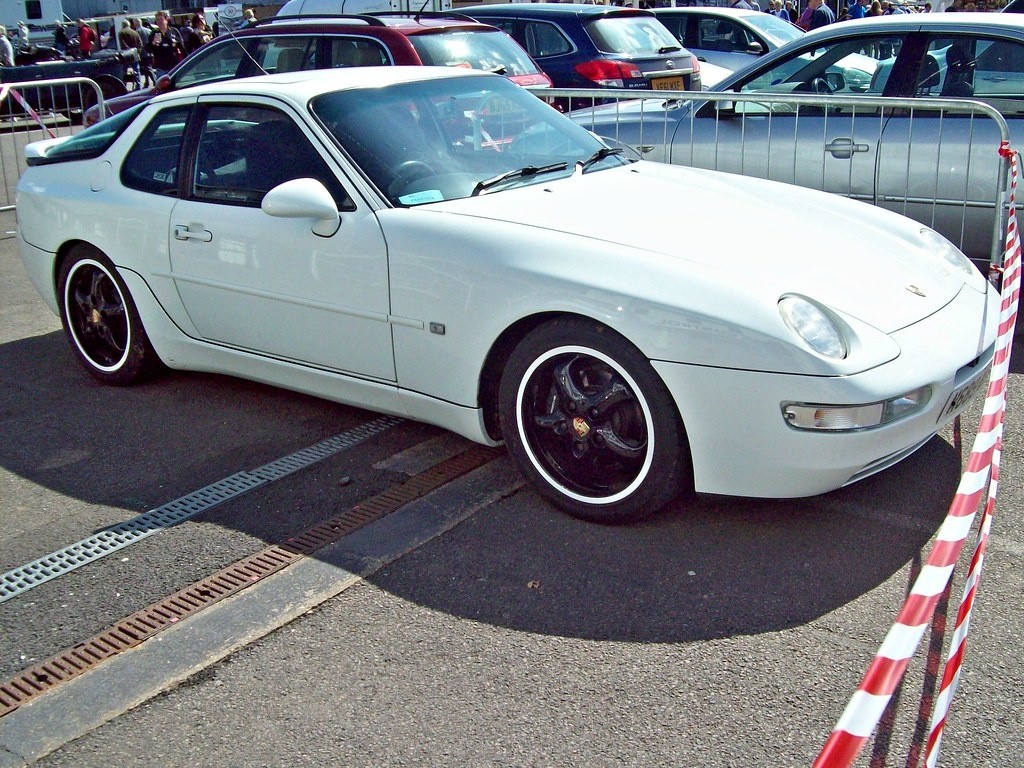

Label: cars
[16,66,1003,522]
[564,12,1024,270]
[0,49,129,123]
[869,0,1024,113]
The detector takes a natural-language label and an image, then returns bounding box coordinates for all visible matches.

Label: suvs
[443,4,703,115]
[82,12,555,152]
[651,7,877,92]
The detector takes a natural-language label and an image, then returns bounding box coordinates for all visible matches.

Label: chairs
[246,121,346,205]
[370,103,429,164]
[353,42,382,66]
[842,58,898,114]
[713,22,734,53]
[334,40,356,67]
[275,49,311,72]
[915,54,940,97]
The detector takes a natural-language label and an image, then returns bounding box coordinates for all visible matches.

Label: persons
[510,1,935,74]
[0,7,264,91]
